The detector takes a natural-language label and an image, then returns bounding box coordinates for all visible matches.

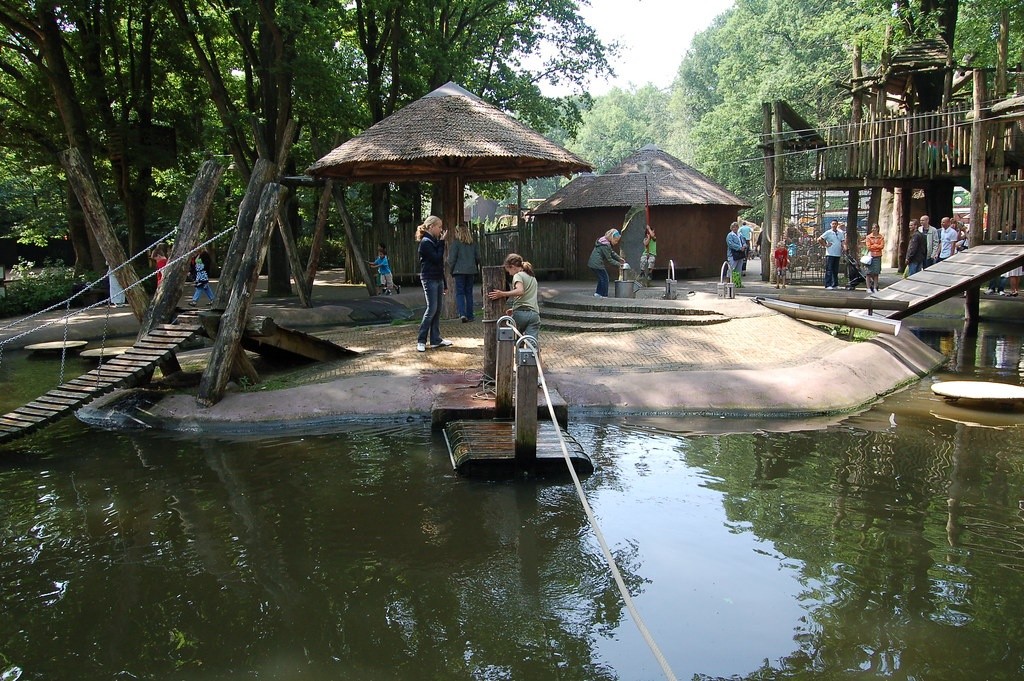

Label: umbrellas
[305,81,593,319]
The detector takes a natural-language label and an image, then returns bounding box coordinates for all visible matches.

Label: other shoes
[873,288,878,293]
[396,285,401,295]
[866,288,872,294]
[640,270,645,277]
[825,285,843,289]
[984,289,994,295]
[1006,291,1018,297]
[206,302,212,306]
[461,316,468,322]
[115,304,123,307]
[188,302,197,307]
[593,292,608,299]
[647,273,653,280]
[998,291,1005,295]
[468,317,476,322]
[782,286,787,290]
[775,286,780,289]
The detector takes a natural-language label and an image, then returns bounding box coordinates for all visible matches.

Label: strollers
[841,251,879,291]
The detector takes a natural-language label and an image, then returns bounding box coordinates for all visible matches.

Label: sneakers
[417,342,425,351]
[430,338,453,348]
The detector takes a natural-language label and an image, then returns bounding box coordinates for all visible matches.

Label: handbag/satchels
[902,265,909,279]
[195,280,207,288]
[860,250,872,266]
[731,248,746,260]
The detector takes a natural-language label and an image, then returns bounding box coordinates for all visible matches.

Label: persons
[775,241,788,289]
[415,215,452,352]
[726,222,749,283]
[866,223,885,294]
[755,230,764,275]
[187,243,214,306]
[818,219,848,289]
[738,222,753,247]
[844,229,861,277]
[365,241,401,296]
[639,224,656,280]
[448,223,481,323]
[587,228,625,298]
[905,214,969,297]
[742,237,747,276]
[984,220,1022,296]
[106,261,126,307]
[783,221,822,258]
[488,253,546,387]
[151,242,173,287]
